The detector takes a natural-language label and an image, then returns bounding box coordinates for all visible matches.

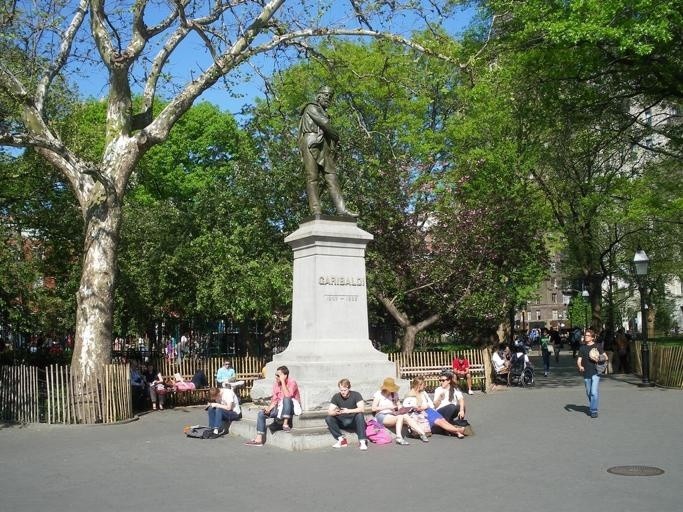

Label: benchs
[399,364,486,393]
[490,359,511,387]
[214,372,261,389]
[162,375,210,393]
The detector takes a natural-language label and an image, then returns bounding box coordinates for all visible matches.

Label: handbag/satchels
[365,423,392,444]
[186,425,225,437]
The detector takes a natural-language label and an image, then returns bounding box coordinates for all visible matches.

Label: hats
[380,378,400,392]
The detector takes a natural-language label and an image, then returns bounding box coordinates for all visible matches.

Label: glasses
[439,380,445,382]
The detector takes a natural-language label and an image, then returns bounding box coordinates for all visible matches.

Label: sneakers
[467,389,474,395]
[591,413,598,418]
[332,427,465,451]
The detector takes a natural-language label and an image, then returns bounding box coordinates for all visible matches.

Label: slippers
[245,440,264,446]
[283,425,291,432]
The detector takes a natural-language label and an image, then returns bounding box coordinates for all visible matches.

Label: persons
[204,381,242,437]
[432,369,467,426]
[450,356,476,391]
[296,85,362,223]
[319,379,374,452]
[487,320,581,384]
[2,333,67,359]
[244,366,301,448]
[403,374,470,440]
[372,378,429,448]
[109,324,244,411]
[576,330,610,418]
